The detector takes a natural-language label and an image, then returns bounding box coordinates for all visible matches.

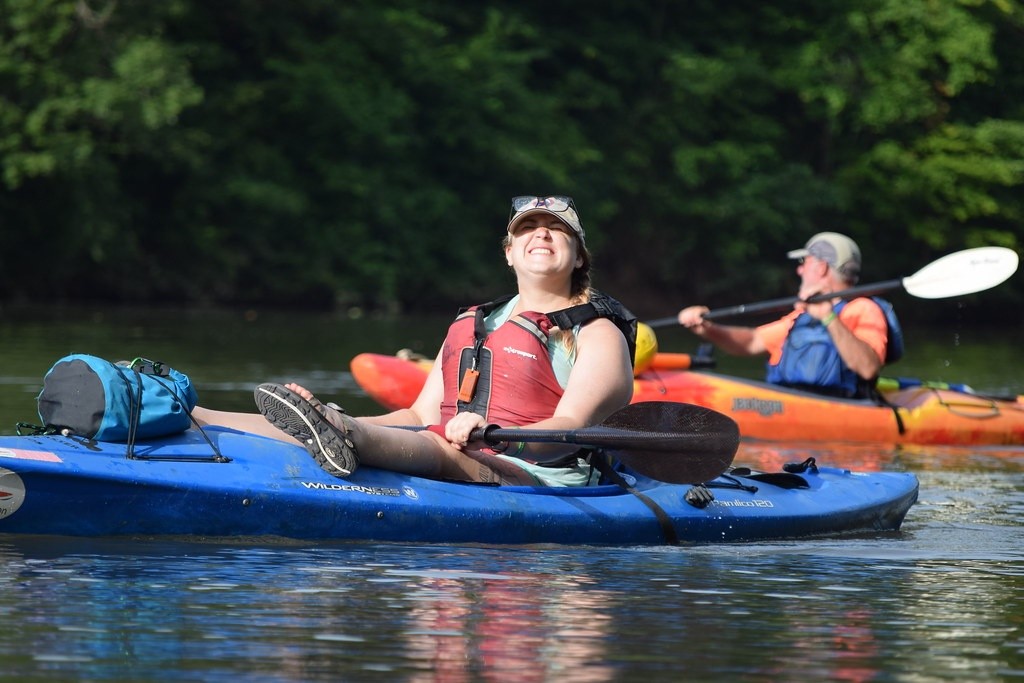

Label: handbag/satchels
[37,356,198,442]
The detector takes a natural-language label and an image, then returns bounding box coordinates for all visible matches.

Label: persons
[678,231,889,401]
[117,196,634,488]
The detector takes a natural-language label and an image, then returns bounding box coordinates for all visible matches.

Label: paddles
[635,239,1019,331]
[371,397,740,493]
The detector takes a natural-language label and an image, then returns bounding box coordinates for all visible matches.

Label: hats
[507,198,585,247]
[787,231,862,283]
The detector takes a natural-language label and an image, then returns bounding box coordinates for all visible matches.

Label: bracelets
[505,425,525,458]
[821,313,836,324]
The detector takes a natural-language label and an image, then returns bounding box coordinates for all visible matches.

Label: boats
[349,348,1024,450]
[0,427,924,546]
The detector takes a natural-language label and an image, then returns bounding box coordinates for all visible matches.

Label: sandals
[254,382,358,477]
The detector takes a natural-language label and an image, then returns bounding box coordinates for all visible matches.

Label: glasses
[508,195,582,226]
